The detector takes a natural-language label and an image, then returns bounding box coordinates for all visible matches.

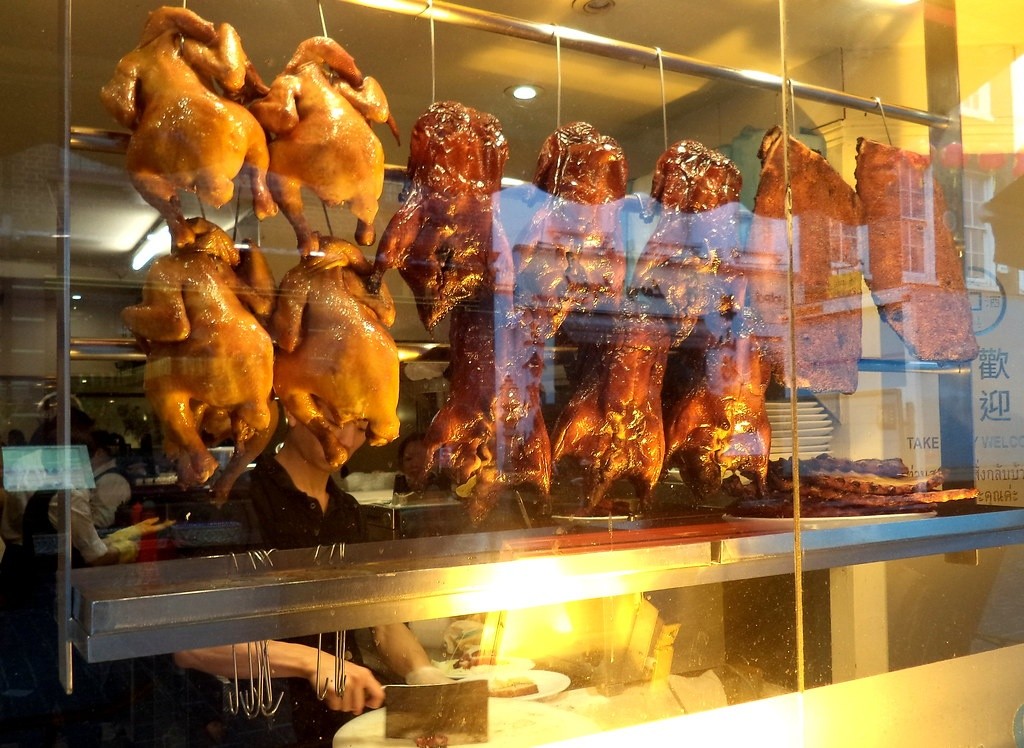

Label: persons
[173,390,460,747]
[88,428,138,526]
[18,426,179,706]
[2,428,35,545]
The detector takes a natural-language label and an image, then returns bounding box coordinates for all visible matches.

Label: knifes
[365,680,489,741]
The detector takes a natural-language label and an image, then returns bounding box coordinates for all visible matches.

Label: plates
[441,658,536,679]
[457,670,571,699]
[669,470,739,481]
[764,400,834,462]
[722,509,937,527]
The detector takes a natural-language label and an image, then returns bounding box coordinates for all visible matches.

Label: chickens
[120,217,280,510]
[247,36,403,261]
[97,6,280,250]
[264,230,401,468]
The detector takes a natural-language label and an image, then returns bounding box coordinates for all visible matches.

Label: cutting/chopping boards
[332,700,592,748]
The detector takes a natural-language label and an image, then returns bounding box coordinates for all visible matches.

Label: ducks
[664,315,774,501]
[626,138,747,349]
[549,287,672,514]
[413,287,551,530]
[366,101,515,335]
[510,120,629,345]
